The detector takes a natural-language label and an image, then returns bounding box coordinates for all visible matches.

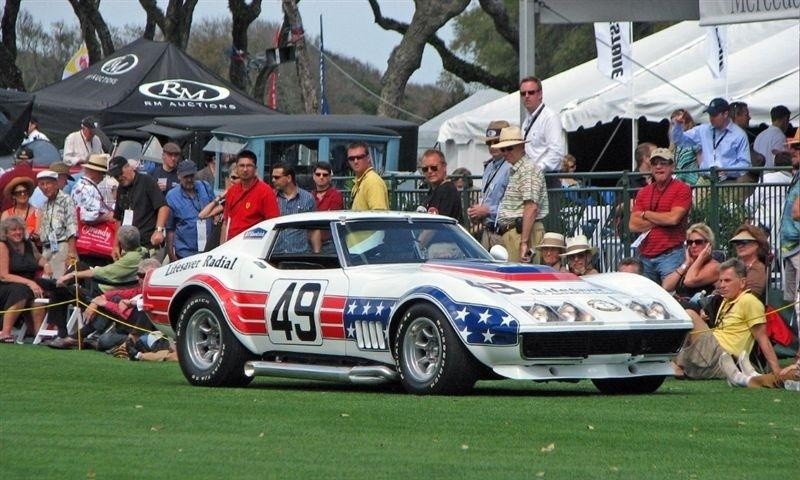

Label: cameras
[524,248,536,261]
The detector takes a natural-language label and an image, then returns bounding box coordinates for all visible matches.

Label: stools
[32,297,83,345]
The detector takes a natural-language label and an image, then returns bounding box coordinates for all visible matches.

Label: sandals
[0,331,15,344]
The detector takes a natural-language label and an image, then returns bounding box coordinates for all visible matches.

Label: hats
[649,147,676,164]
[3,176,35,201]
[784,126,800,144]
[727,229,760,243]
[81,117,97,129]
[702,98,729,114]
[36,169,60,180]
[489,127,532,149]
[176,159,198,178]
[109,156,128,178]
[479,120,510,142]
[535,231,566,249]
[80,153,108,172]
[163,142,182,155]
[557,234,599,257]
[49,162,70,174]
[16,148,34,159]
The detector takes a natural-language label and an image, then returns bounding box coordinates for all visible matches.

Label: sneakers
[738,349,755,375]
[125,339,140,361]
[44,336,84,349]
[718,351,741,389]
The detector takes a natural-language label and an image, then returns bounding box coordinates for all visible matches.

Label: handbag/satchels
[74,205,124,260]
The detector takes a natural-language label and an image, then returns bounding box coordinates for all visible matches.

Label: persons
[345,142,389,263]
[1,111,343,361]
[418,76,799,395]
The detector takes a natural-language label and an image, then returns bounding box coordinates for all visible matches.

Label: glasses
[485,140,499,145]
[567,163,576,169]
[314,172,330,177]
[137,271,146,279]
[499,143,522,153]
[421,160,441,173]
[11,190,30,195]
[347,154,367,161]
[230,175,241,180]
[790,143,800,150]
[270,174,288,179]
[686,238,708,246]
[450,175,467,181]
[519,88,541,96]
[733,240,757,245]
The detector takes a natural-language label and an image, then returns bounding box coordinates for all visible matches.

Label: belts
[497,219,544,237]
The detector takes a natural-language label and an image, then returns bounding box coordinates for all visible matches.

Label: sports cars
[143,210,690,395]
